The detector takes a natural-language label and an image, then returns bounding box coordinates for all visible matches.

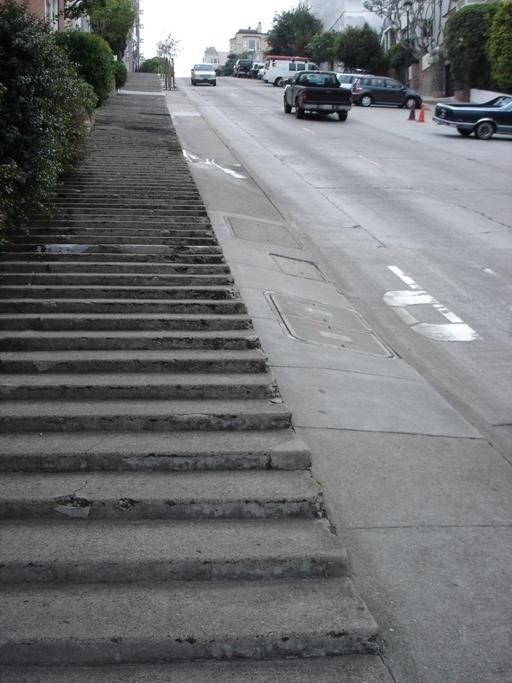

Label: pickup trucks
[433,94,512,139]
[282,69,353,121]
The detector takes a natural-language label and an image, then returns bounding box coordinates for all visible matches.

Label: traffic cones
[416,102,424,122]
[407,99,416,120]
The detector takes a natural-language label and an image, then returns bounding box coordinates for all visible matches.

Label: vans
[263,55,322,85]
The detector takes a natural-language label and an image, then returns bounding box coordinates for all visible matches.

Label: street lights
[403,0,414,87]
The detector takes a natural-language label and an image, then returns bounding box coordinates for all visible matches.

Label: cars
[330,69,392,104]
[351,75,422,108]
[232,58,265,78]
[191,62,217,86]
